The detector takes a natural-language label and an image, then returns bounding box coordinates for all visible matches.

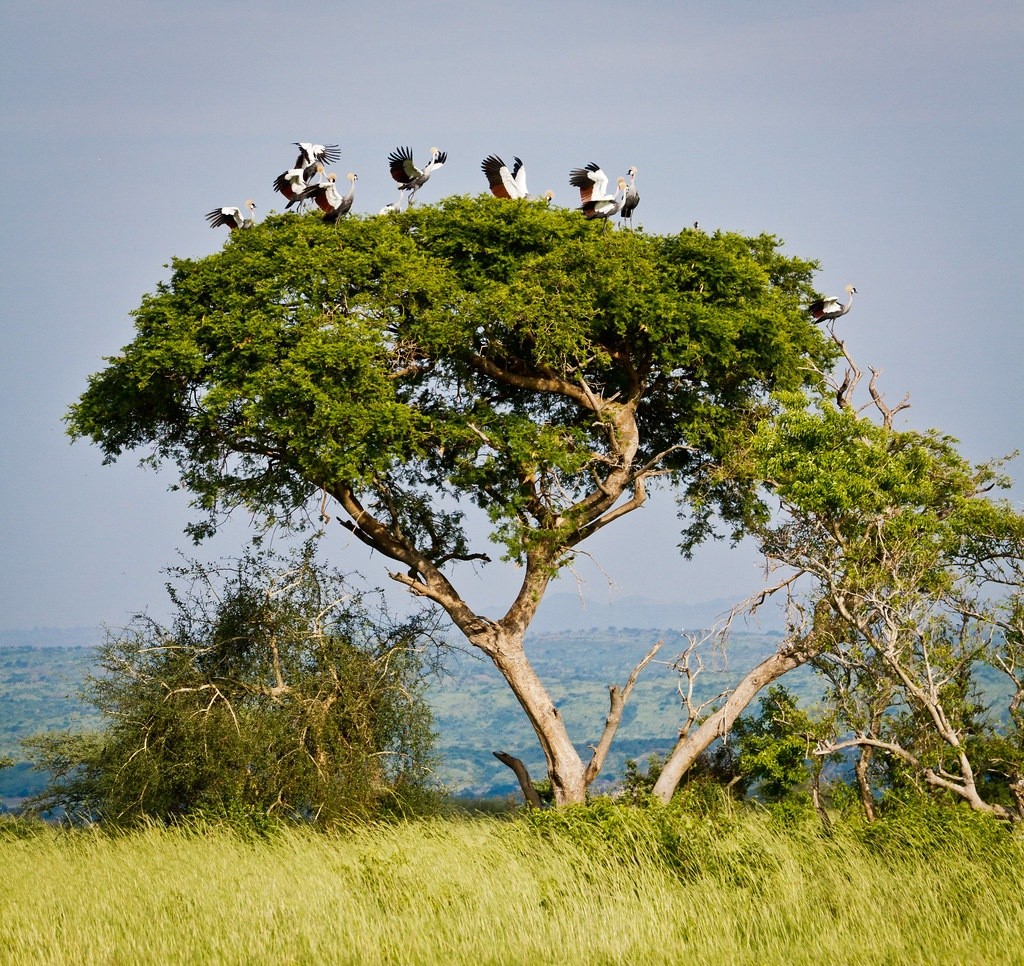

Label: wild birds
[804,280,857,331]
[199,128,642,237]
